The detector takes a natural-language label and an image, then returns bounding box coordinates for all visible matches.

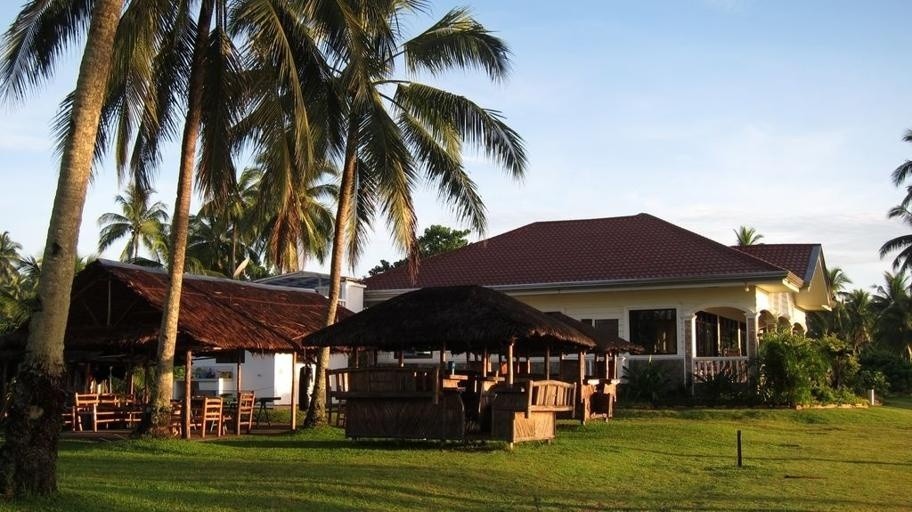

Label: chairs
[73,390,255,441]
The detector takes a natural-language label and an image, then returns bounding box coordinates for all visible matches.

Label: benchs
[494,379,576,419]
